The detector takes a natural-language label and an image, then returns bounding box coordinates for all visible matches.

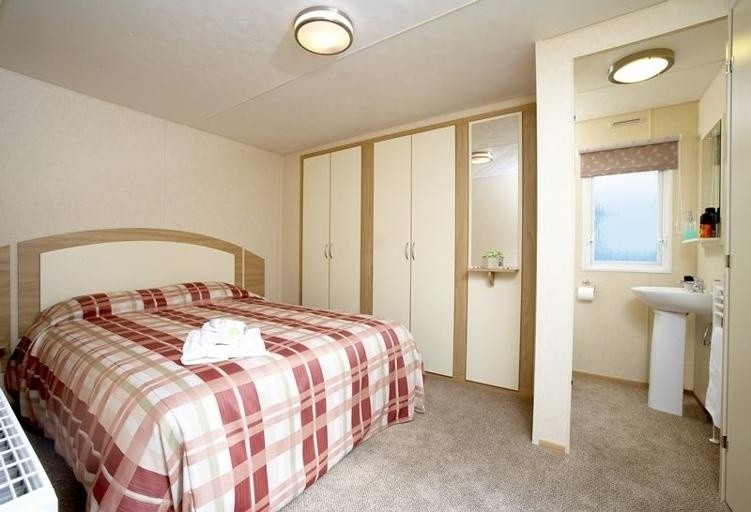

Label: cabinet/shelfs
[300,125,456,379]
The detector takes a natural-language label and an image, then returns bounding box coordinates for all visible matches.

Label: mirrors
[701,119,721,214]
[469,111,522,269]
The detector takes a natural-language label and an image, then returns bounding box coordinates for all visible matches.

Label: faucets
[677,278,703,292]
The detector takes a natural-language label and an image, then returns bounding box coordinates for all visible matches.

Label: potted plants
[479,247,504,267]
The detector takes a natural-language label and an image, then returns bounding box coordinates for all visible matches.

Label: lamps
[607,48,676,84]
[293,6,354,55]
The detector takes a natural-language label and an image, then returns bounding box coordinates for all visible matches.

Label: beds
[16,227,414,512]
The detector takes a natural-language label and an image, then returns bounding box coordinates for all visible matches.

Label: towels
[704,327,724,431]
[181,318,273,366]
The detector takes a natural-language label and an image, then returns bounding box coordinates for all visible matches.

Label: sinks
[631,286,713,317]
